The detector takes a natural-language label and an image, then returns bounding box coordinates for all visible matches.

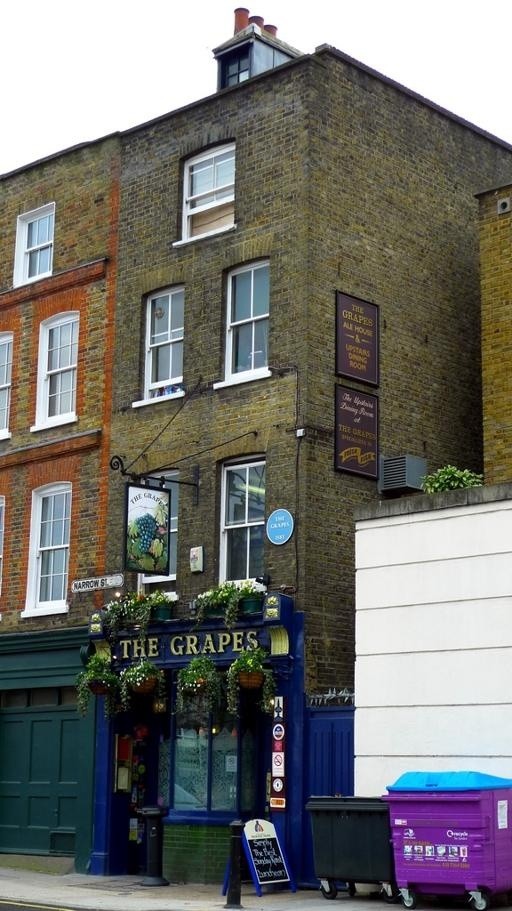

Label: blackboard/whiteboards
[241,819,292,885]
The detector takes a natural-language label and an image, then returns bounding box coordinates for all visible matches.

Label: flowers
[223,580,267,628]
[150,588,172,606]
[226,646,275,717]
[74,654,118,721]
[175,655,224,722]
[102,590,150,646]
[192,581,241,630]
[118,656,166,714]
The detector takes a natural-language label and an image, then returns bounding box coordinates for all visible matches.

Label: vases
[243,598,263,614]
[153,606,172,619]
[88,680,110,694]
[239,671,263,690]
[132,677,156,693]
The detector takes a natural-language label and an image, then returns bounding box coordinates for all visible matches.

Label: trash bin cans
[305,771,512,911]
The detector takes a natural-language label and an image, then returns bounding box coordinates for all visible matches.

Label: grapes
[134,513,157,552]
